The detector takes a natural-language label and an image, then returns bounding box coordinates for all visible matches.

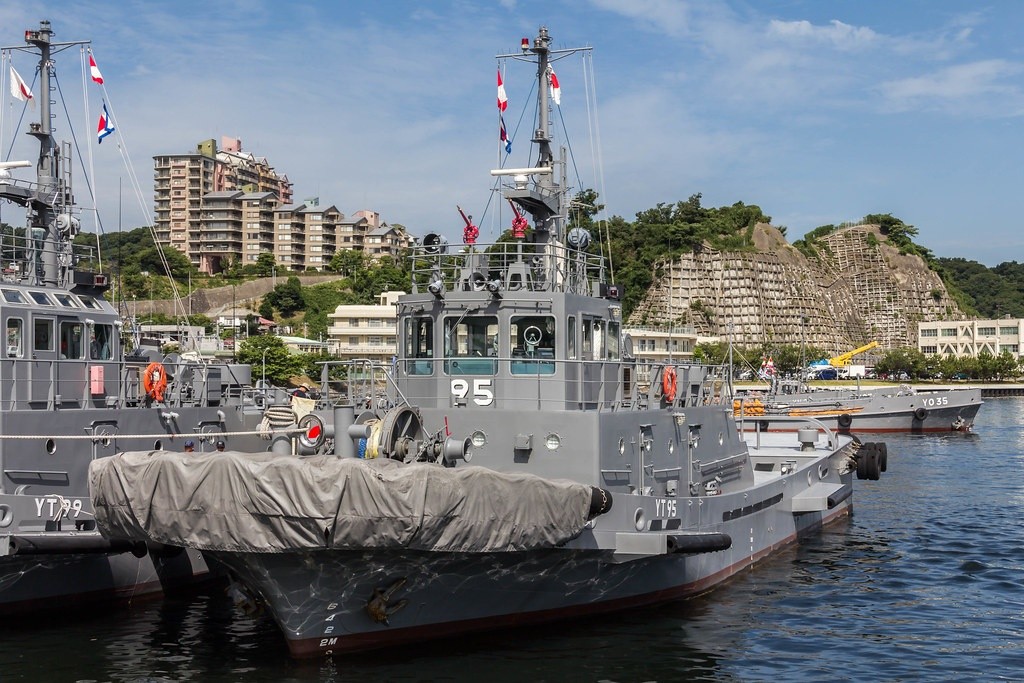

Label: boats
[0,20,987,656]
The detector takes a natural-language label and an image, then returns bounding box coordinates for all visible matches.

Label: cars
[741,341,972,380]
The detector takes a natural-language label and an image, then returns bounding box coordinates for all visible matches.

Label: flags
[10,65,36,111]
[497,68,512,154]
[89,54,104,85]
[98,100,115,144]
[547,59,561,105]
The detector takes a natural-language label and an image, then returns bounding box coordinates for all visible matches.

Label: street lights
[262,347,270,407]
[132,294,136,350]
[137,321,153,345]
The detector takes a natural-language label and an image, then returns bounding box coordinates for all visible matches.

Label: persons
[290,383,310,403]
[184,440,195,452]
[215,441,225,452]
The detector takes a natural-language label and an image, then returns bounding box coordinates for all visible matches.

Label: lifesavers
[839,414,852,427]
[144,363,167,397]
[663,367,678,401]
[915,408,928,421]
[755,420,769,431]
[857,442,888,482]
